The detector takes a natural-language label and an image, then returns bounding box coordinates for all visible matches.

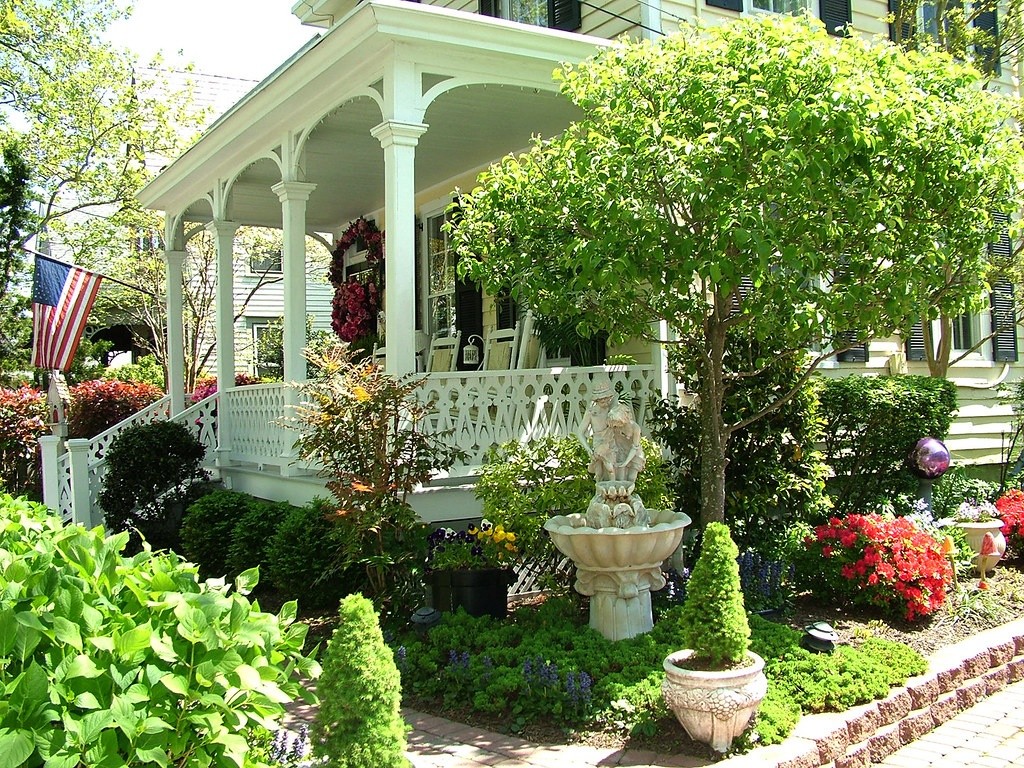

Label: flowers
[424,523,519,572]
[994,488,1024,543]
[325,214,385,344]
[803,510,954,623]
[952,496,1000,523]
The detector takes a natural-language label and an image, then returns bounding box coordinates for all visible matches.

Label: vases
[951,516,1006,573]
[422,569,519,620]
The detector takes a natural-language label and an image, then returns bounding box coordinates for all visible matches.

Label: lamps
[463,334,486,370]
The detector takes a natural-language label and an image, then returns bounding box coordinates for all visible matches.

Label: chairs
[429,309,543,428]
[426,331,461,389]
[471,321,521,397]
[373,342,386,372]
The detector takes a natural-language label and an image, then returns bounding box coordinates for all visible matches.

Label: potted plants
[663,521,768,754]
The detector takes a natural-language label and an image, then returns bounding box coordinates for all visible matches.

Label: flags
[31,252,104,373]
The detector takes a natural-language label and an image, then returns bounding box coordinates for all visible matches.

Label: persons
[578,381,645,482]
